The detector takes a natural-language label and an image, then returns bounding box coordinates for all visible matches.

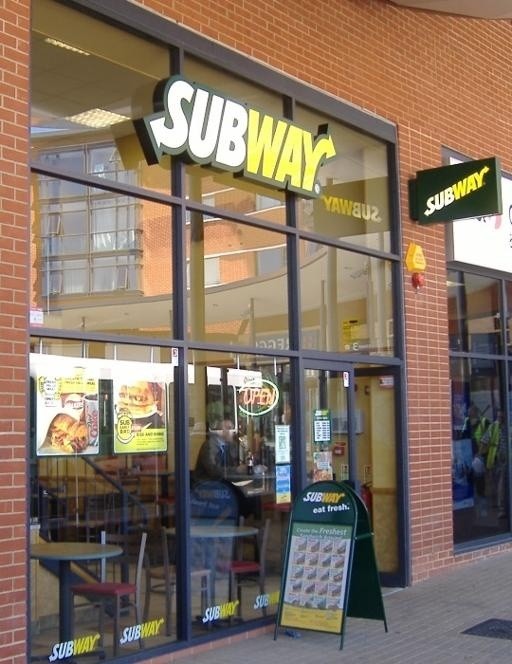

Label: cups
[83,392,99,447]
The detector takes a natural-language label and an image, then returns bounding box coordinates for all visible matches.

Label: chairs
[139,524,213,637]
[71,530,147,660]
[214,517,271,628]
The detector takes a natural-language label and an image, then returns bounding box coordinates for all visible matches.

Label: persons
[464,405,493,460]
[195,419,252,531]
[471,407,507,520]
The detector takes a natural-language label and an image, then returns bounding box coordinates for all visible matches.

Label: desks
[30,542,123,664]
[167,525,258,629]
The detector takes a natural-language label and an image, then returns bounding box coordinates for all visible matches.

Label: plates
[116,404,158,419]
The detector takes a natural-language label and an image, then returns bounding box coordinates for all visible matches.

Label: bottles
[247,451,253,475]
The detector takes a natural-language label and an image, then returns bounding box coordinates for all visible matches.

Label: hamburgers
[45,414,89,453]
[119,384,154,416]
